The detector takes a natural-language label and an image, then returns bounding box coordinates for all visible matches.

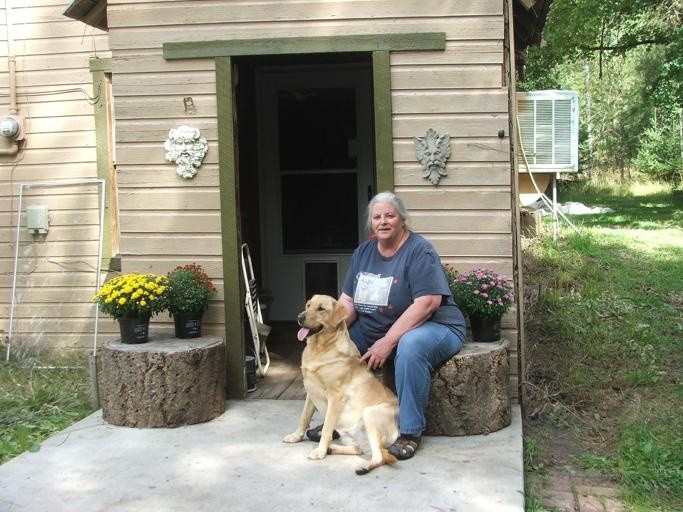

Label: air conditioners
[516,88,579,176]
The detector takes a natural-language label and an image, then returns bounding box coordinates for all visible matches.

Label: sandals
[386,430,424,459]
[305,421,340,442]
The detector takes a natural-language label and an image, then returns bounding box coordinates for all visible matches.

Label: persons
[163,125,208,180]
[412,129,452,188]
[304,190,468,461]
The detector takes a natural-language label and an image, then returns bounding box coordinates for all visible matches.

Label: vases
[468,310,503,343]
[116,314,151,346]
[170,310,204,338]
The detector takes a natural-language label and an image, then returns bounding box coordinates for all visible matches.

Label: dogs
[283,295,402,475]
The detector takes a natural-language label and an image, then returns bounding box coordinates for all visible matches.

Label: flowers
[165,263,218,314]
[450,268,516,319]
[91,275,175,318]
